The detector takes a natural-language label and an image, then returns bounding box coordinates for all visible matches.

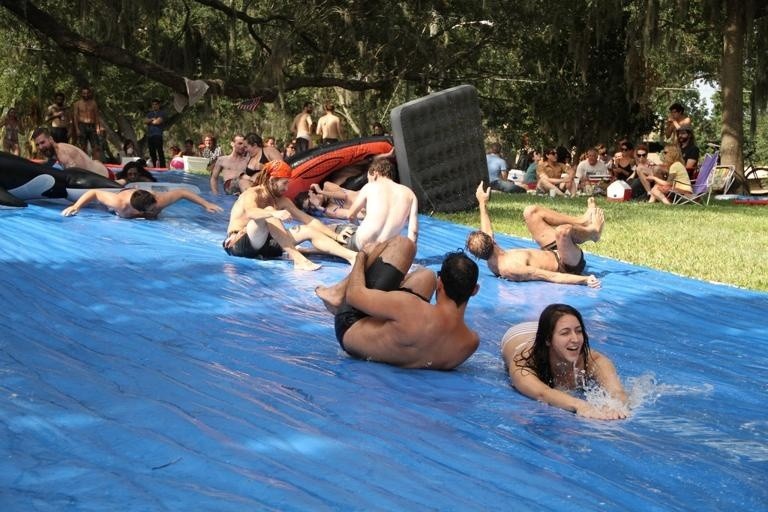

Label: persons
[170,145,181,156]
[177,140,196,156]
[649,167,669,205]
[611,143,634,181]
[514,136,534,169]
[291,102,313,155]
[26,128,126,187]
[264,137,275,146]
[294,180,367,220]
[61,187,222,220]
[313,235,479,370]
[594,144,610,166]
[0,107,24,155]
[466,180,605,289]
[293,157,418,255]
[485,142,526,192]
[143,99,165,169]
[316,103,343,147]
[280,139,297,158]
[523,149,541,190]
[211,135,253,196]
[647,144,691,204]
[241,133,282,184]
[73,89,101,159]
[45,93,69,163]
[574,149,609,195]
[534,147,576,199]
[630,146,656,198]
[372,123,388,137]
[501,305,628,420]
[118,138,137,165]
[613,139,634,157]
[665,103,690,145]
[222,159,380,270]
[671,129,699,201]
[198,133,221,161]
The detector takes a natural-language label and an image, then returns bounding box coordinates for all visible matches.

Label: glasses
[436,271,441,278]
[664,151,670,154]
[636,152,648,158]
[621,147,630,153]
[204,139,213,141]
[599,149,609,156]
[287,146,296,149]
[307,199,313,213]
[535,151,538,154]
[670,110,678,113]
[548,151,558,156]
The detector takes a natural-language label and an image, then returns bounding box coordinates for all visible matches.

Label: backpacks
[516,149,531,169]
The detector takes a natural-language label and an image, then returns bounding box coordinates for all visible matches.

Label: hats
[678,125,694,132]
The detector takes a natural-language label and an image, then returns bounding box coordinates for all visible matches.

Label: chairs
[668,152,719,206]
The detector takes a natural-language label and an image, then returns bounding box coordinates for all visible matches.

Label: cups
[549,189,556,199]
